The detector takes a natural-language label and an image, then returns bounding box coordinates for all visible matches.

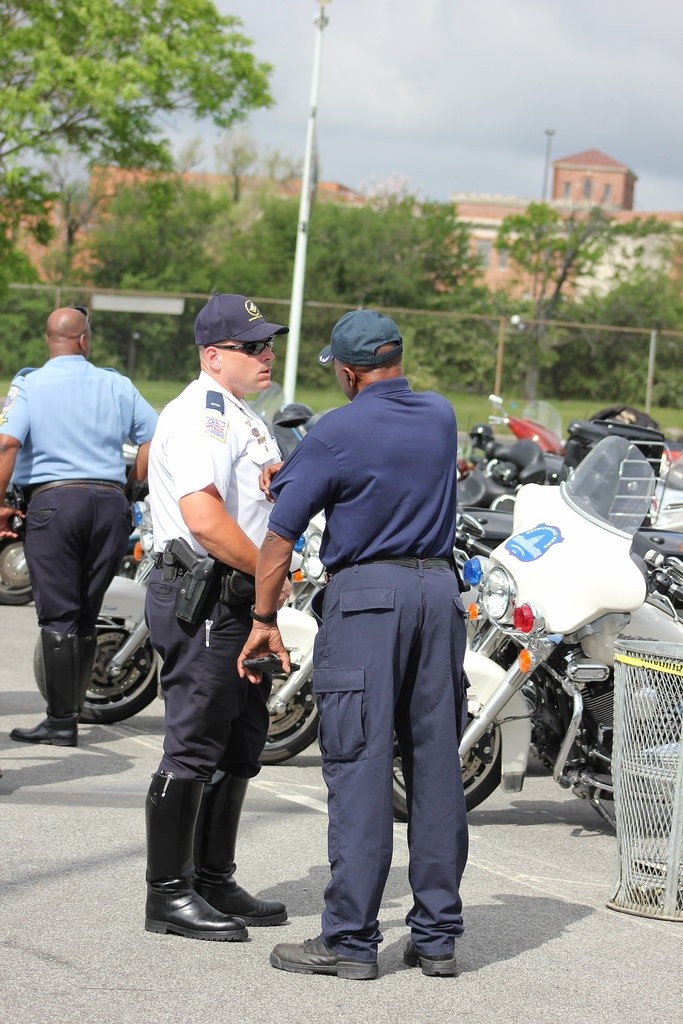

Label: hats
[317,310,404,365]
[194,294,290,347]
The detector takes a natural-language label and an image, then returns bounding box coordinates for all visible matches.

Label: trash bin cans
[604,639,683,923]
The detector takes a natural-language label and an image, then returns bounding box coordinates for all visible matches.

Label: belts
[23,477,126,501]
[359,558,453,569]
[153,555,164,567]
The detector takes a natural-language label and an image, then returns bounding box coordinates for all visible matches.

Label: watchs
[250,604,277,622]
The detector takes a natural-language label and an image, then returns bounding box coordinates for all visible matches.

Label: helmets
[470,425,494,440]
[272,403,314,427]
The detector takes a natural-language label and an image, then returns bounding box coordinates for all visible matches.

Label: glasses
[70,306,89,334]
[205,338,274,354]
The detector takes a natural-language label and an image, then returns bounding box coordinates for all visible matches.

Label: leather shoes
[404,938,458,976]
[270,935,378,979]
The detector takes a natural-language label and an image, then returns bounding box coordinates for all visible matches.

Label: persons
[236,309,472,979]
[0,306,158,746]
[143,292,292,940]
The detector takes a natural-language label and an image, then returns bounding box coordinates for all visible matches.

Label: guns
[8,487,24,530]
[169,537,218,625]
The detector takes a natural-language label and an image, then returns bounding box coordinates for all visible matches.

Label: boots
[191,765,288,927]
[145,774,248,941]
[9,627,99,746]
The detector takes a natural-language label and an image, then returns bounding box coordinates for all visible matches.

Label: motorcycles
[393,434,683,832]
[0,381,683,766]
